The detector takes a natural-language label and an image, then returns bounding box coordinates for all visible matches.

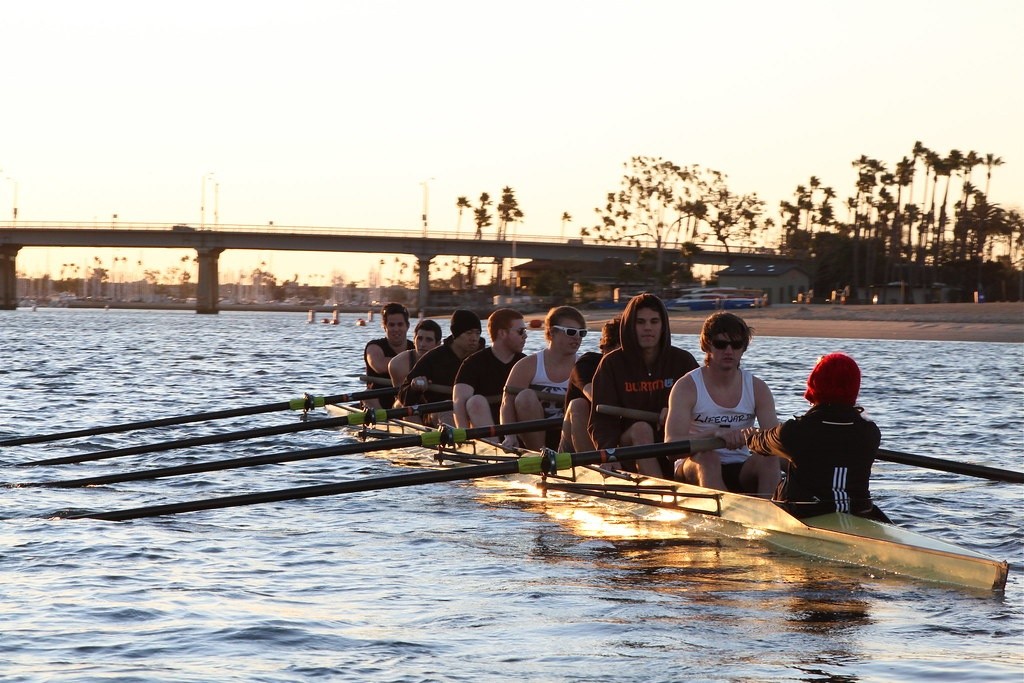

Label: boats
[665,294,762,311]
[321,390,1010,594]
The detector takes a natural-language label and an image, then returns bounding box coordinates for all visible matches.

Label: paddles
[596,398,1024,481]
[0,387,749,520]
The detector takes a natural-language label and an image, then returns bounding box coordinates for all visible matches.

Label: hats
[450,310,481,338]
[804,353,861,405]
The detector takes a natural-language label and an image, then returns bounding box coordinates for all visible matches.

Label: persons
[398,310,486,429]
[740,354,882,518]
[557,315,621,453]
[500,306,586,451]
[452,308,529,442]
[588,294,700,480]
[664,313,781,498]
[388,320,442,425]
[364,303,415,407]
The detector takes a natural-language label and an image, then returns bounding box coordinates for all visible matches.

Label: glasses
[552,325,587,338]
[711,340,745,350]
[506,328,527,335]
